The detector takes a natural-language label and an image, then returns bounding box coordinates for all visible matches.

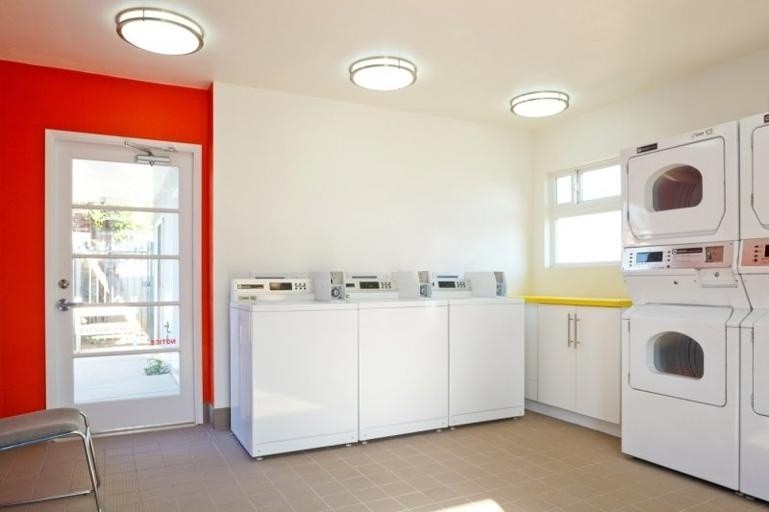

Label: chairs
[0,405,101,511]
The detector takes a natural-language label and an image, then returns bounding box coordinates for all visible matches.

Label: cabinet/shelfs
[525,303,535,414]
[536,303,621,439]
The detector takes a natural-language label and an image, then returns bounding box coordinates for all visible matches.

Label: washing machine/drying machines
[229,272,359,460]
[357,267,449,446]
[620,111,769,502]
[447,270,525,430]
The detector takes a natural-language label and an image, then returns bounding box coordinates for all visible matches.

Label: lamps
[509,88,570,120]
[349,56,419,91]
[115,7,205,57]
